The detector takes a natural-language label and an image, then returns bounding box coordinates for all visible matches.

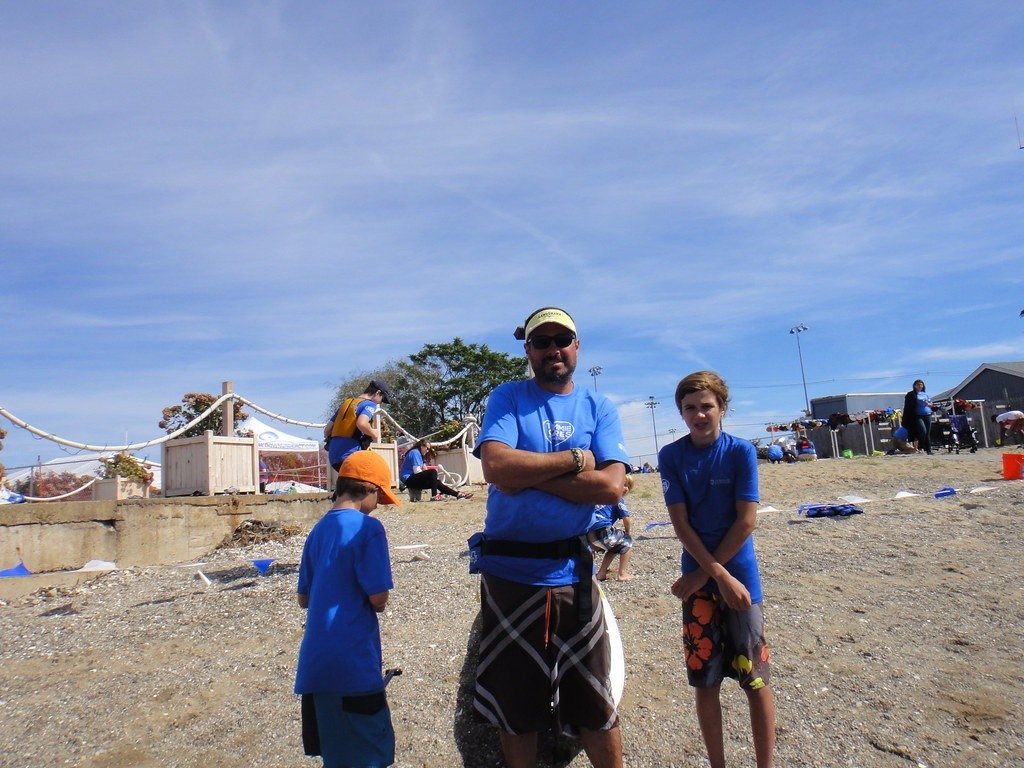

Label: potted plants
[431,416,487,485]
[327,420,400,491]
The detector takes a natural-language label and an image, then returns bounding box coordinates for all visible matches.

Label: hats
[372,380,391,404]
[338,450,402,508]
[514,309,577,338]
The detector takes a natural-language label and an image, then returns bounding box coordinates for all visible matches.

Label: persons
[883,426,918,454]
[904,379,935,455]
[324,380,392,472]
[400,439,473,501]
[588,473,634,582]
[658,372,775,768]
[796,435,817,461]
[991,410,1024,449]
[258,455,268,494]
[766,443,783,464]
[293,447,404,768]
[467,308,633,768]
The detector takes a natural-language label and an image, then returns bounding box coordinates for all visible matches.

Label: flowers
[158,392,255,436]
[95,453,154,486]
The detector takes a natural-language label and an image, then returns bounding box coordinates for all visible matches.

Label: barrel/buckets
[1002,453,1024,480]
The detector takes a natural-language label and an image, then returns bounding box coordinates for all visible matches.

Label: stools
[409,487,421,501]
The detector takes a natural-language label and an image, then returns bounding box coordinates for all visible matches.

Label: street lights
[789,322,810,417]
[644,395,660,466]
[588,366,602,392]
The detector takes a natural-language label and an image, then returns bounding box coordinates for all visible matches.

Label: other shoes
[430,494,446,501]
[457,491,473,500]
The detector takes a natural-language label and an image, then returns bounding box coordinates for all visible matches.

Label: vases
[92,475,149,500]
[160,430,261,495]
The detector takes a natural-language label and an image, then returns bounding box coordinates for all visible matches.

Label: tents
[232,418,322,493]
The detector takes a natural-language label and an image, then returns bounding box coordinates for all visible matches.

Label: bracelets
[577,447,586,472]
[569,448,581,471]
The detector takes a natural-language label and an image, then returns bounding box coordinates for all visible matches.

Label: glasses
[426,447,430,450]
[528,333,575,349]
